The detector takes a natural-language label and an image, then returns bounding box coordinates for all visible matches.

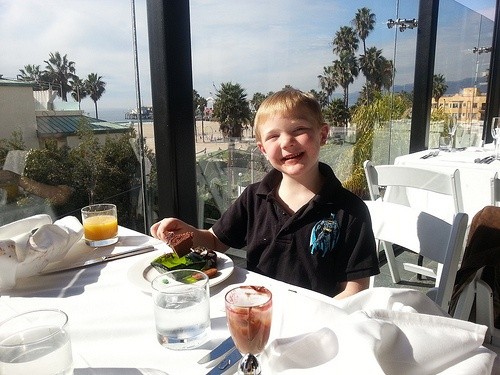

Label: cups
[0,308,74,375]
[151,269,211,350]
[81,204,119,248]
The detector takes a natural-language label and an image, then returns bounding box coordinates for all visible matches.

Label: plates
[127,249,234,295]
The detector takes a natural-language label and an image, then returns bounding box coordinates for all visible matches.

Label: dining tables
[0,226,497,375]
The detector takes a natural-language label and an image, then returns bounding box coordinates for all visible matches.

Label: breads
[164,232,196,258]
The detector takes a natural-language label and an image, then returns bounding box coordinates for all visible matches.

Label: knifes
[38,245,154,275]
[205,349,243,375]
[197,337,235,364]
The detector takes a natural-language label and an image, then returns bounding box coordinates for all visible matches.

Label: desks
[383,147,500,280]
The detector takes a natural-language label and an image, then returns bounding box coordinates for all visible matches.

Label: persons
[148,88,380,300]
[0,153,79,223]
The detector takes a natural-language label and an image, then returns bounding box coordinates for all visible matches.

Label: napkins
[258,287,488,375]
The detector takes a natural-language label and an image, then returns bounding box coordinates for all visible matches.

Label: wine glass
[224,285,272,375]
[491,117,500,155]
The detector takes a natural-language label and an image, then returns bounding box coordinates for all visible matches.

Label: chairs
[360,200,468,315]
[453,279,500,354]
[363,159,464,282]
